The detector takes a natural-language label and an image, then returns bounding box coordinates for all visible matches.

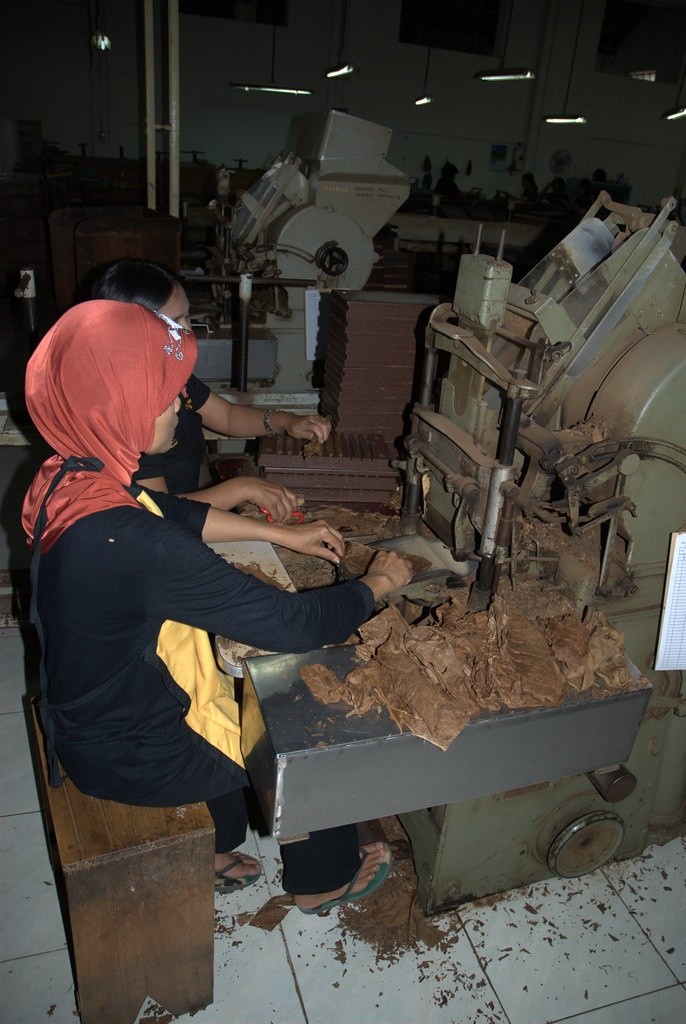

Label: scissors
[258,504,328,526]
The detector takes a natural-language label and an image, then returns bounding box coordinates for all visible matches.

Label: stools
[24,689,218,1024]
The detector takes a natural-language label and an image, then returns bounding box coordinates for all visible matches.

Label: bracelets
[263,408,283,435]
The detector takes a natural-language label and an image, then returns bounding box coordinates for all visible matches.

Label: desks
[50,206,182,312]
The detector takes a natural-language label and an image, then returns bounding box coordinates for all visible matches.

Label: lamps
[229,26,315,96]
[415,46,435,106]
[661,65,686,122]
[541,0,590,124]
[324,0,355,79]
[474,0,537,83]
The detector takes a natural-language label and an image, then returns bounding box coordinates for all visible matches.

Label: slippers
[213,851,262,890]
[298,841,391,915]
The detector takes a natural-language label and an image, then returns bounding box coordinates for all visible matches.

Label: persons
[91,257,332,769]
[433,164,460,195]
[570,179,595,207]
[22,299,415,914]
[521,173,539,202]
[540,177,569,203]
